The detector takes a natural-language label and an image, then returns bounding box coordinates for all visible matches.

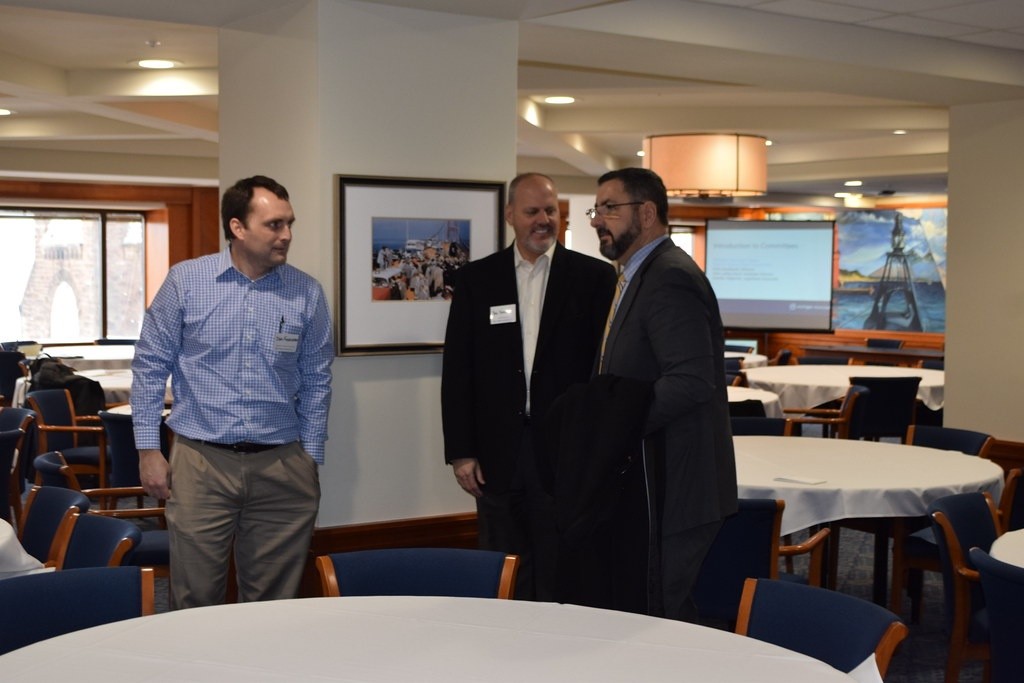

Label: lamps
[639,133,770,200]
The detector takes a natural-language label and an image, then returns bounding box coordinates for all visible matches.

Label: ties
[598,273,627,375]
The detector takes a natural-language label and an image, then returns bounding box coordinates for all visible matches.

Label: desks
[987,529,1024,567]
[724,352,768,369]
[108,401,172,420]
[732,429,1001,609]
[74,364,182,401]
[726,384,783,421]
[0,595,857,682]
[2,519,58,578]
[742,363,947,441]
[37,340,150,367]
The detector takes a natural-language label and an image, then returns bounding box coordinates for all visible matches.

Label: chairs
[0,322,1024,682]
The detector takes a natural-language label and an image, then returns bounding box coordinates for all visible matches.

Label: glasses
[585,200,645,223]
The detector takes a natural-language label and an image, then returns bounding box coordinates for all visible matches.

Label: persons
[378,240,466,300]
[590,167,738,623]
[129,177,334,609]
[441,172,615,576]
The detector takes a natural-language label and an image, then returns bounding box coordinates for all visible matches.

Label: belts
[181,433,283,456]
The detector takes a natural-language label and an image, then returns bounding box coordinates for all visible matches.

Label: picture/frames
[328,176,509,358]
